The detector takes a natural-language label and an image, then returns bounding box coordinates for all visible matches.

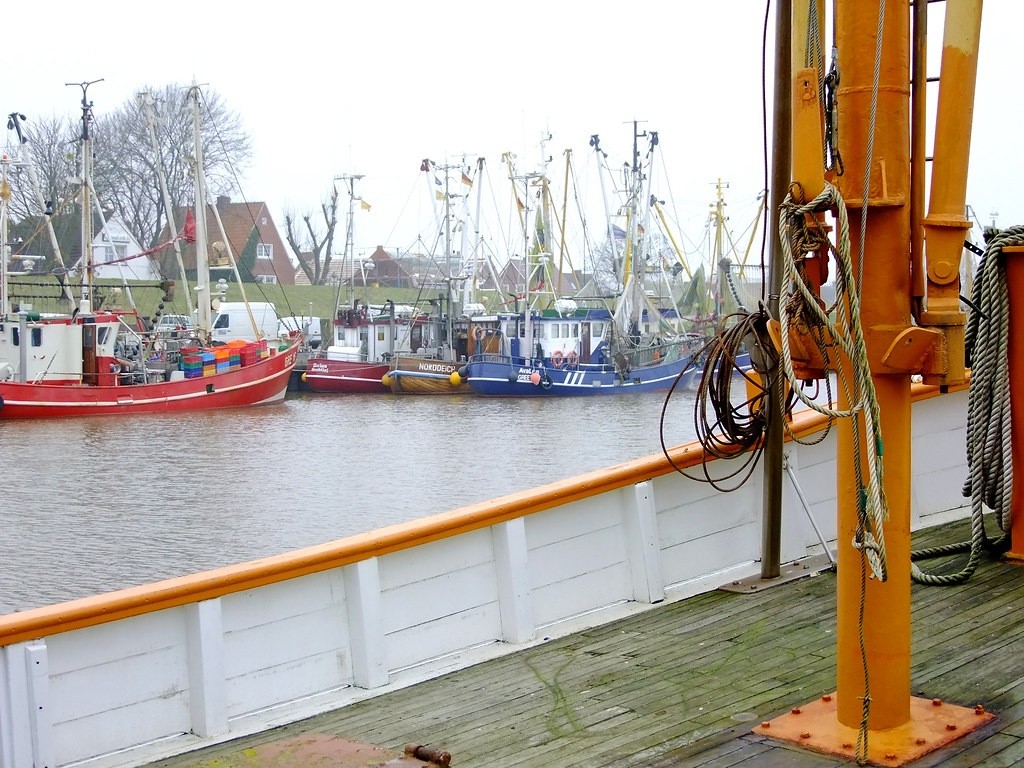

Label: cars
[153,314,193,331]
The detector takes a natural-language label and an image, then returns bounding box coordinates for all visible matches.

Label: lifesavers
[567,350,578,368]
[539,374,553,390]
[553,350,564,368]
[472,325,485,341]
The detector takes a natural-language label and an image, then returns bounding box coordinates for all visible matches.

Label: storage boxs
[178,338,269,379]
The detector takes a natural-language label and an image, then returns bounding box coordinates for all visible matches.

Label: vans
[278,316,321,349]
[186,301,278,346]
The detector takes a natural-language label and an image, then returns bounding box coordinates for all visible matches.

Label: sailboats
[458,120,708,398]
[301,157,480,392]
[380,131,604,396]
[693,178,754,379]
[0,74,304,420]
